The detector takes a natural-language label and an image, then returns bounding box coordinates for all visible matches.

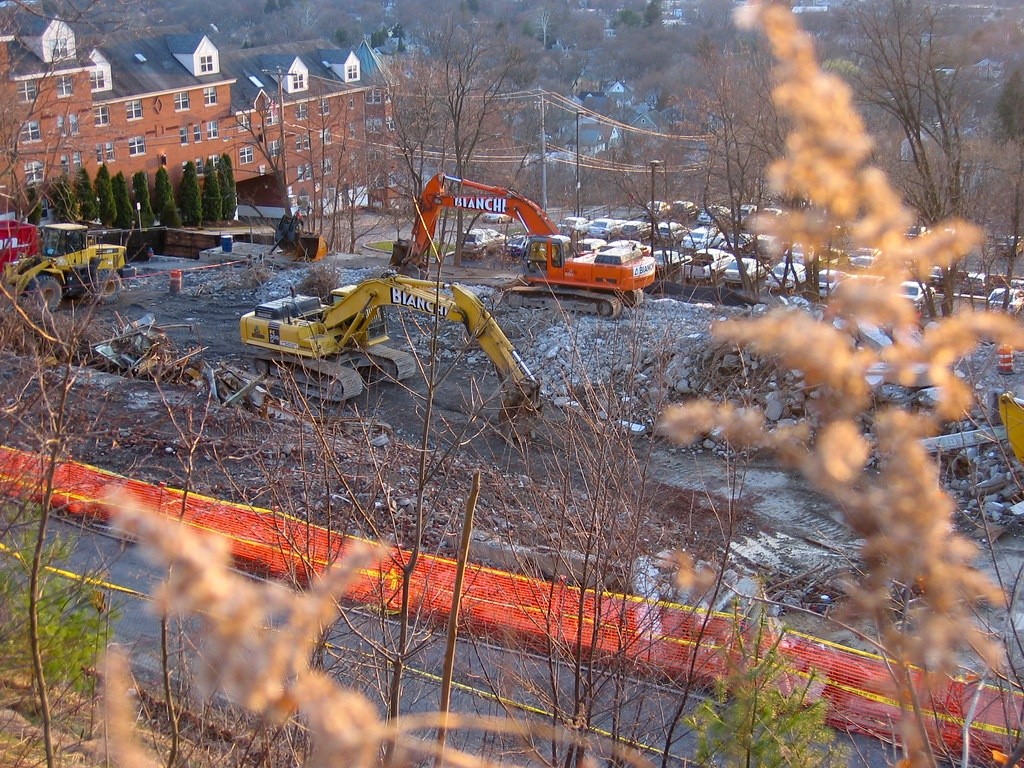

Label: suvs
[985,288,1023,318]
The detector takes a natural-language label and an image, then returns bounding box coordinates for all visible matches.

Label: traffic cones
[996,341,1014,374]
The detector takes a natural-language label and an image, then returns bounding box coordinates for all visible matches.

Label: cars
[559,214,589,236]
[587,216,622,239]
[814,269,933,317]
[723,258,766,285]
[646,197,1024,260]
[682,247,735,284]
[483,208,512,224]
[768,261,808,291]
[506,234,679,279]
[656,219,1024,294]
[621,220,650,240]
[458,225,505,255]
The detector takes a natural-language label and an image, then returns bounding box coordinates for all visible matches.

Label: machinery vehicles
[240,270,544,445]
[4,224,127,316]
[391,173,656,321]
